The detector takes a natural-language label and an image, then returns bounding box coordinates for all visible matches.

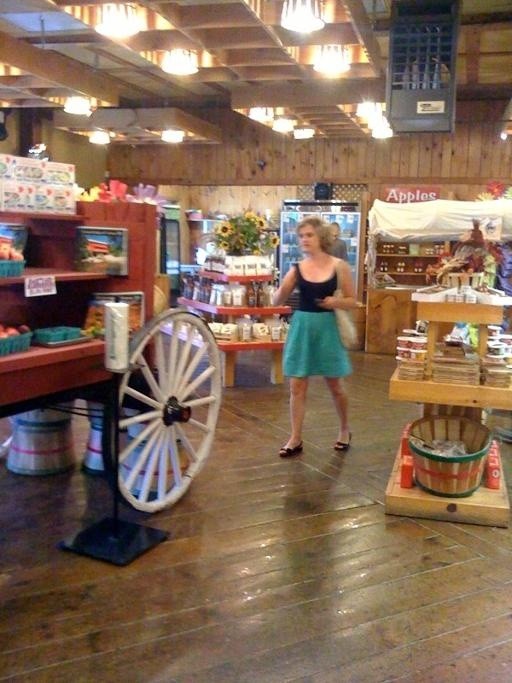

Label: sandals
[280,440,303,455]
[335,432,352,450]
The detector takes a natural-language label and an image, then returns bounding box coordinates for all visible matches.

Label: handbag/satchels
[334,290,357,349]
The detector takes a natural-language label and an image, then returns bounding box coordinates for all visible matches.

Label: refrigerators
[278,211,360,306]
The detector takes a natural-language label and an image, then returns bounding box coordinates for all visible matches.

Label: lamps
[88,130,113,145]
[271,117,294,133]
[278,0,327,34]
[249,107,275,121]
[162,50,200,76]
[92,2,141,42]
[159,128,185,144]
[354,99,395,144]
[292,129,315,141]
[62,95,91,115]
[312,45,355,78]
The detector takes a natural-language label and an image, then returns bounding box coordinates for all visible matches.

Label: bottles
[256,279,266,308]
[247,278,256,308]
[184,273,244,307]
[238,314,253,342]
[269,313,282,343]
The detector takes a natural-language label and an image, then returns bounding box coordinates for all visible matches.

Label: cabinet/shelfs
[384,301,512,529]
[159,269,294,388]
[0,201,156,407]
[363,217,450,355]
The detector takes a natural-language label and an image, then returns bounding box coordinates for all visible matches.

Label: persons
[271,215,360,460]
[326,219,350,266]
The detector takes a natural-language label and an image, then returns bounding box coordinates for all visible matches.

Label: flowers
[212,210,280,256]
